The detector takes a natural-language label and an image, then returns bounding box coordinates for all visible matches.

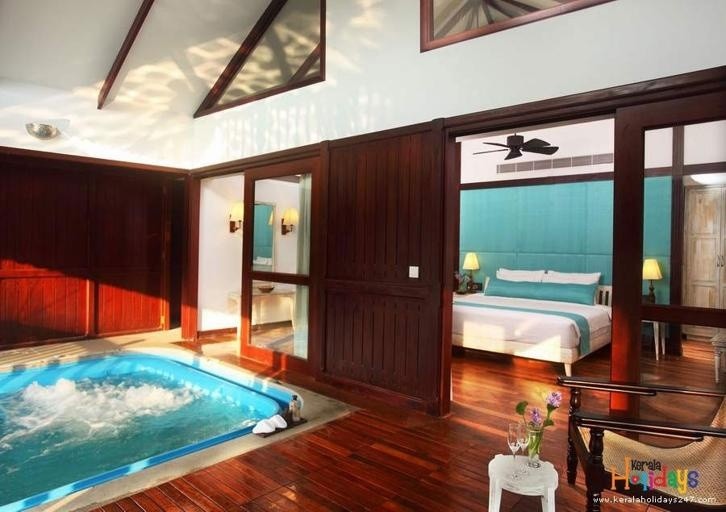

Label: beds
[452,267,613,377]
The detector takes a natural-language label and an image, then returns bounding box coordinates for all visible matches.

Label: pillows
[496,267,544,287]
[544,270,602,286]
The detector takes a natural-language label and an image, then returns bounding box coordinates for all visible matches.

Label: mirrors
[253,201,277,274]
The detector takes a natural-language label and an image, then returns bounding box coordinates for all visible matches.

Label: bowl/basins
[257,286,275,293]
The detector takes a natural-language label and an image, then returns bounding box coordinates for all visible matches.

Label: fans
[471,134,559,160]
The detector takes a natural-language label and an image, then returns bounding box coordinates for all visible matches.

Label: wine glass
[507,424,531,482]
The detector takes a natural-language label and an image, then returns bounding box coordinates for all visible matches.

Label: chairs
[553,377,726,512]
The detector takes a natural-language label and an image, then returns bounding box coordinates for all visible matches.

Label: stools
[642,320,666,361]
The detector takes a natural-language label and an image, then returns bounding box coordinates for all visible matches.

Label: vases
[528,427,544,468]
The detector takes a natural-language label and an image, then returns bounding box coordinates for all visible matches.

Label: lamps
[229,202,244,231]
[463,253,480,293]
[281,205,299,233]
[642,260,663,304]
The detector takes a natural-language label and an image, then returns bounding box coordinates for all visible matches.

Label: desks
[486,453,559,512]
[709,328,726,384]
[228,285,296,331]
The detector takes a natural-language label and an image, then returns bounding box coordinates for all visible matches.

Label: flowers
[516,390,561,467]
